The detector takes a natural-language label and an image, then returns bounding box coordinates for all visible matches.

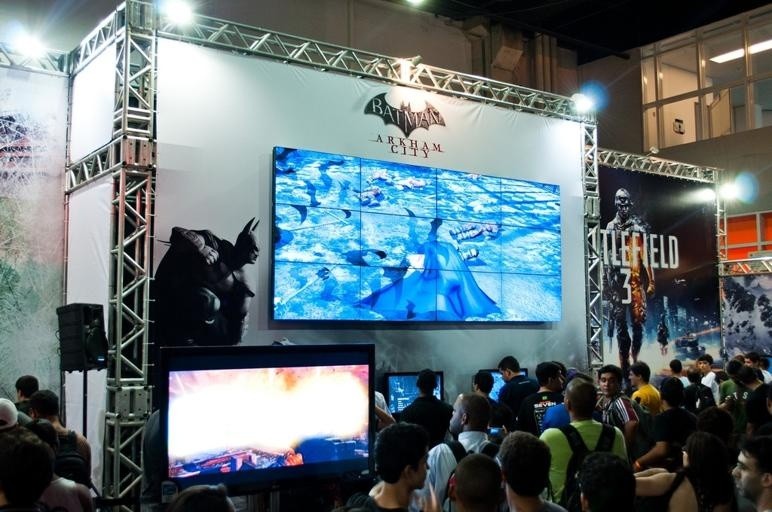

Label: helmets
[615,188,633,206]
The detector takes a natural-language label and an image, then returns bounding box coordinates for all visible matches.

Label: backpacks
[442,440,500,512]
[556,423,616,512]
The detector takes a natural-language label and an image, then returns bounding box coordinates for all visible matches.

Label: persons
[343,355,637,512]
[165,483,236,511]
[0,374,93,512]
[601,188,654,369]
[598,356,771,512]
[154,218,261,347]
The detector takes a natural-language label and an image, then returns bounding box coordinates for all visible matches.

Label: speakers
[56,303,108,373]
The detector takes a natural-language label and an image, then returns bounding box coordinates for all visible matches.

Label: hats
[661,377,683,392]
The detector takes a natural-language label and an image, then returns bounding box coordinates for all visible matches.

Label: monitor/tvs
[385,371,443,414]
[160,344,376,496]
[482,369,528,402]
